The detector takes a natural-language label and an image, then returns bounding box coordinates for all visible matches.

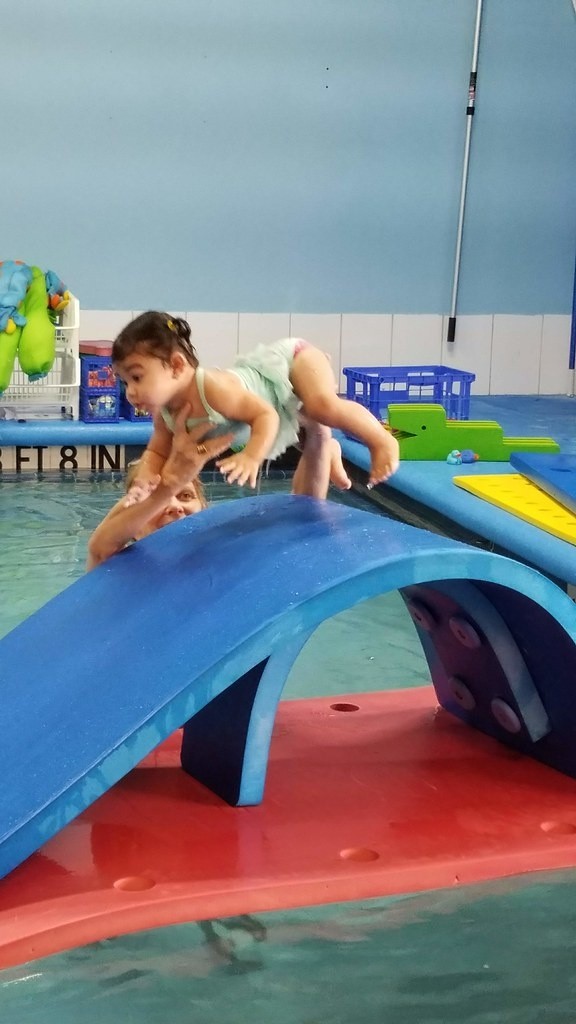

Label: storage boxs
[0,289,155,424]
[342,366,475,445]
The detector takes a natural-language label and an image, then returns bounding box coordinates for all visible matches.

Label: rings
[197,444,207,455]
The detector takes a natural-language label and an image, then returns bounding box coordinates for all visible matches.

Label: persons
[86,456,209,573]
[111,310,400,509]
